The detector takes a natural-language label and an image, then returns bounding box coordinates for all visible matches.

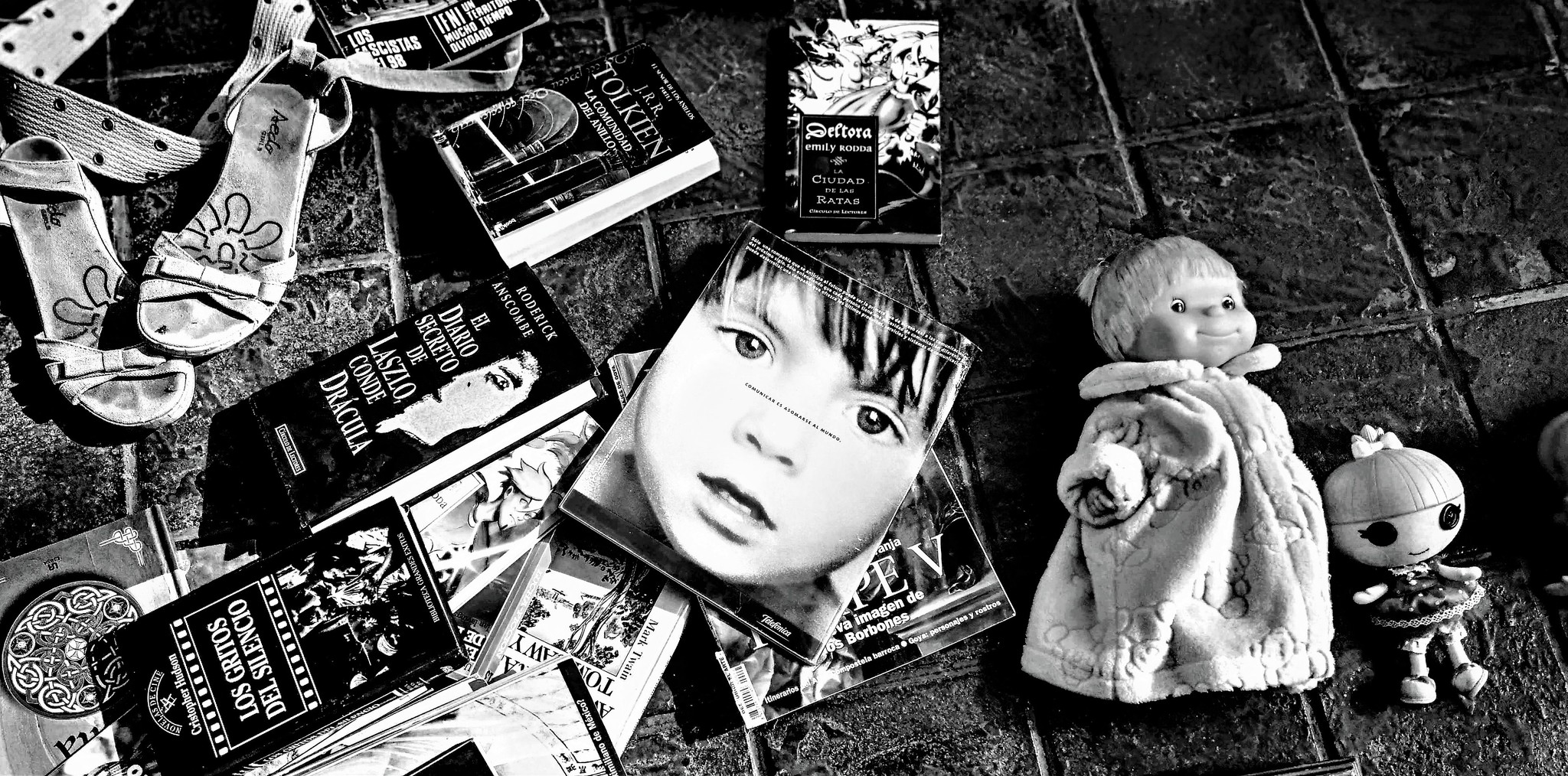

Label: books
[0,15,1016,776]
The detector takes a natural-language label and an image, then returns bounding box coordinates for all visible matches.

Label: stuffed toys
[1322,424,1492,706]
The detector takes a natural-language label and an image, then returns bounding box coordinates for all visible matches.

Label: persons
[596,223,971,644]
[373,342,577,451]
[1018,232,1339,704]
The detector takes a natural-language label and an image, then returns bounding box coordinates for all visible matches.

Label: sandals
[0,137,193,429]
[134,35,354,358]
